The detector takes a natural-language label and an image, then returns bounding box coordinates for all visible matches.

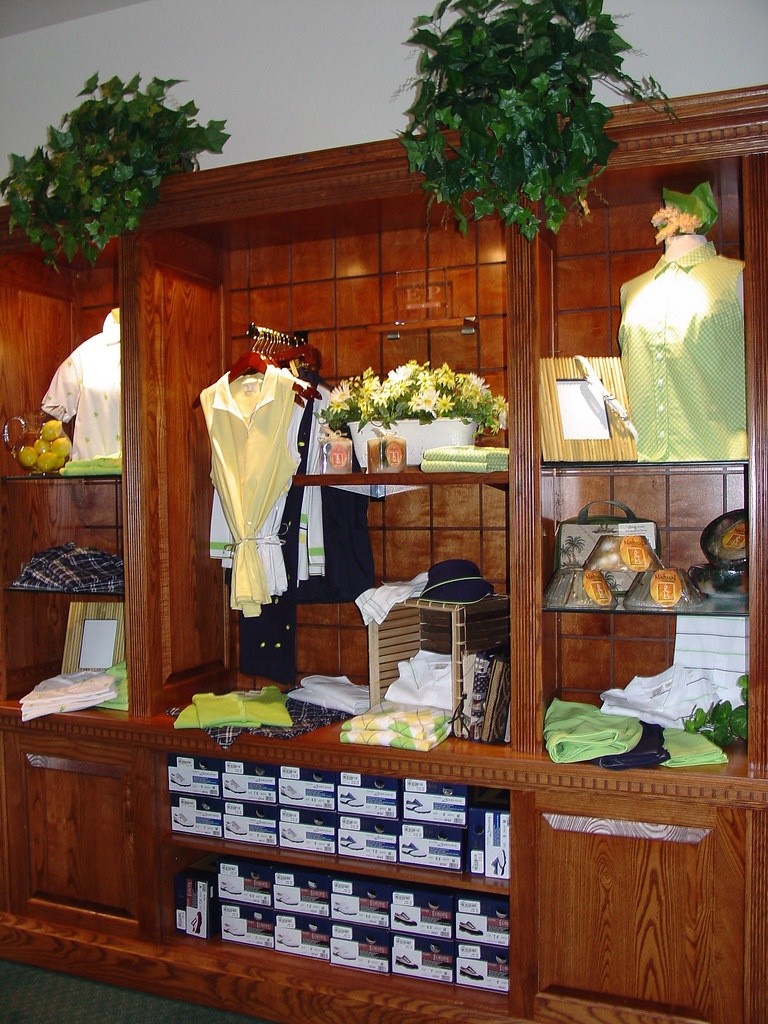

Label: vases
[347,417,477,470]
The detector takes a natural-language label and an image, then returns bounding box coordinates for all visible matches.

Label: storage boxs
[403,778,470,827]
[222,800,278,846]
[390,890,510,993]
[277,805,337,854]
[331,877,393,927]
[169,792,223,838]
[222,759,279,804]
[337,770,403,818]
[278,765,337,810]
[174,856,331,961]
[336,813,398,862]
[466,794,511,880]
[329,922,388,973]
[398,823,466,873]
[165,754,221,799]
[367,594,511,738]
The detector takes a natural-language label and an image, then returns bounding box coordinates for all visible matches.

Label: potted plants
[392,0,680,241]
[0,72,233,273]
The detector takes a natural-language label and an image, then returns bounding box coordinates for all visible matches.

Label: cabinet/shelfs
[0,84,768,1024]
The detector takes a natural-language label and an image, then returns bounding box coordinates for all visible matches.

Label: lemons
[18,420,70,474]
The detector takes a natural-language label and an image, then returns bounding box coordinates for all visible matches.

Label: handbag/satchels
[552,499,662,596]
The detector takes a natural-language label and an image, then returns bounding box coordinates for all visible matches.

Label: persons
[617,232,747,463]
[43,308,123,460]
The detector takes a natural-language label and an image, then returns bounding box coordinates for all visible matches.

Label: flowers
[650,205,703,245]
[315,360,509,439]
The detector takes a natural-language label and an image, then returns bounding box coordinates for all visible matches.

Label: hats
[419,559,495,605]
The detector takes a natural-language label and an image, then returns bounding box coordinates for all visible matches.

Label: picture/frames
[540,356,638,465]
[61,602,125,675]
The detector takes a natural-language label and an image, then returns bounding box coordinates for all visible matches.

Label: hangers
[192,326,333,408]
